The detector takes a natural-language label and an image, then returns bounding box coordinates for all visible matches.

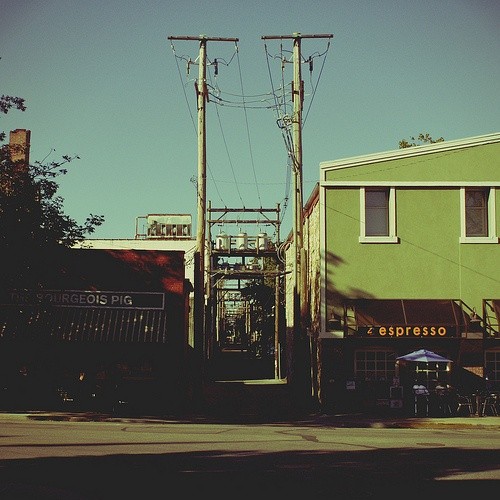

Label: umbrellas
[395,348,453,362]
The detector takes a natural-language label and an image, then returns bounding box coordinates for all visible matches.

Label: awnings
[343,298,500,338]
[0,306,167,343]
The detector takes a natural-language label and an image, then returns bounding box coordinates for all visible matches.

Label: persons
[413,379,453,415]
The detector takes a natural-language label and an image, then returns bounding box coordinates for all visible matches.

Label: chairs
[415,394,499,417]
